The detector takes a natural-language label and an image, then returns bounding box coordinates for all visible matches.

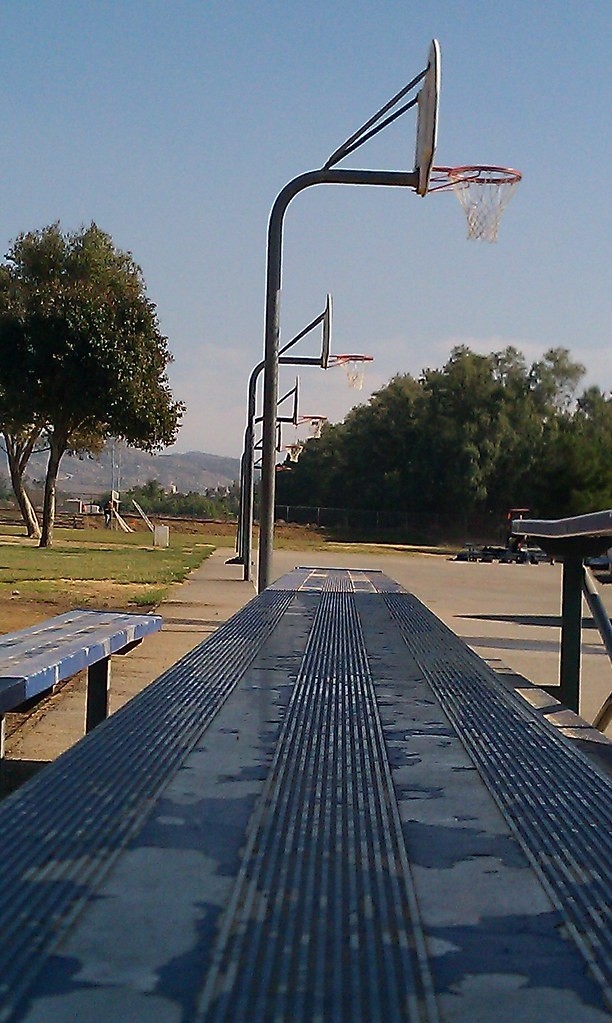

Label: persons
[103,501,112,528]
[511,537,527,564]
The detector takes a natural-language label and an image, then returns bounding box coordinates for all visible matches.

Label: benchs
[0,607,162,757]
